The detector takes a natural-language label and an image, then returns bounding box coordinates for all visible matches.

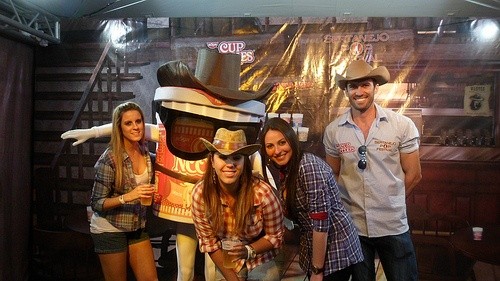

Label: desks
[449,223,500,281]
[65,212,171,281]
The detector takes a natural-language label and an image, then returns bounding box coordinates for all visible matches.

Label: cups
[140,182,155,206]
[87,206,94,221]
[221,239,242,269]
[472,226,483,240]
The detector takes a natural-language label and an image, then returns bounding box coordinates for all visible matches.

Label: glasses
[358,145,367,169]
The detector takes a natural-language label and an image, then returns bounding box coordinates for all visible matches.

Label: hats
[335,59,390,90]
[200,128,262,157]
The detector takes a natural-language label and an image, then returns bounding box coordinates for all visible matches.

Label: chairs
[32,227,101,281]
[406,207,479,281]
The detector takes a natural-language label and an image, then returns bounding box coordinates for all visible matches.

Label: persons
[259,117,365,281]
[323,60,422,281]
[89,102,158,281]
[191,127,285,281]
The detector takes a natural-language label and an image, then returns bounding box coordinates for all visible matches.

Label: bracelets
[119,195,126,204]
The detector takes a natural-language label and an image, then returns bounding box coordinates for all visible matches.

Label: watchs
[312,266,324,275]
[245,245,256,259]
